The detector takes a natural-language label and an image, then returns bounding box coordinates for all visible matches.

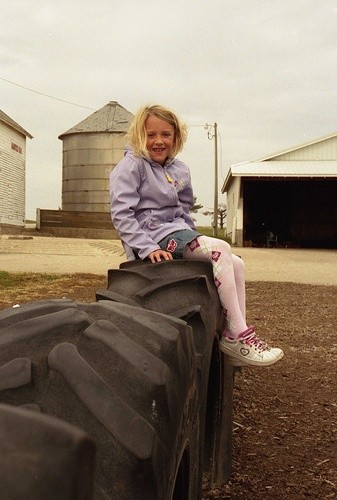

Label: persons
[108,101,285,368]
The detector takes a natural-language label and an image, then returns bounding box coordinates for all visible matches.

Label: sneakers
[221,325,284,367]
